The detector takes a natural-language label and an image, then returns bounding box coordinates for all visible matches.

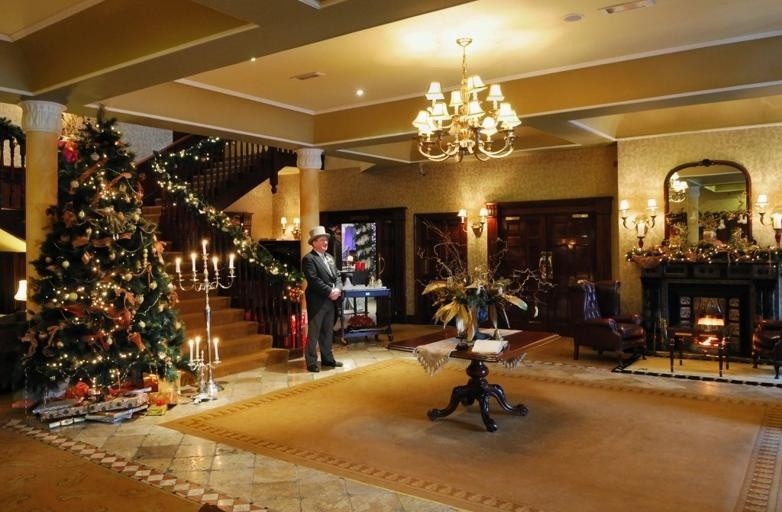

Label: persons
[302,226,343,372]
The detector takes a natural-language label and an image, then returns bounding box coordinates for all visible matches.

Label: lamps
[619,199,658,248]
[14,280,26,303]
[755,195,782,248]
[280,217,301,240]
[669,172,689,203]
[457,208,488,238]
[411,39,523,162]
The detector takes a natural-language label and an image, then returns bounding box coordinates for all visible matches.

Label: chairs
[569,281,647,369]
[751,318,782,379]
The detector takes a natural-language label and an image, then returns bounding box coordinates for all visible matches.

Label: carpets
[612,352,782,390]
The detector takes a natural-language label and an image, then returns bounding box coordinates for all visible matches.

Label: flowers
[416,216,558,328]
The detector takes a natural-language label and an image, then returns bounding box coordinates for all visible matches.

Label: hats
[308,226,331,244]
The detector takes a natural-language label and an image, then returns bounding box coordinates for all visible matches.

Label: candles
[228,253,235,268]
[188,339,195,364]
[212,256,219,270]
[212,337,220,361]
[190,252,197,271]
[201,240,208,255]
[175,258,181,272]
[194,336,202,359]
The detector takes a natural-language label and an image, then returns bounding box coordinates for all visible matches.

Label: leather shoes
[322,360,342,366]
[308,365,319,372]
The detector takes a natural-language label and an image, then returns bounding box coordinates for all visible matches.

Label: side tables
[666,323,730,377]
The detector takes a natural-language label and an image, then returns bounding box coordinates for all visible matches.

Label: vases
[454,304,476,342]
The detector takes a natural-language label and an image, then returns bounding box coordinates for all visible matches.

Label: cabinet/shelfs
[332,288,393,344]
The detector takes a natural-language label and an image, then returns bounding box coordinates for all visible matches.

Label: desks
[411,337,528,432]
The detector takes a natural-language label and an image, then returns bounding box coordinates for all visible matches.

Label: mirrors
[663,159,752,246]
